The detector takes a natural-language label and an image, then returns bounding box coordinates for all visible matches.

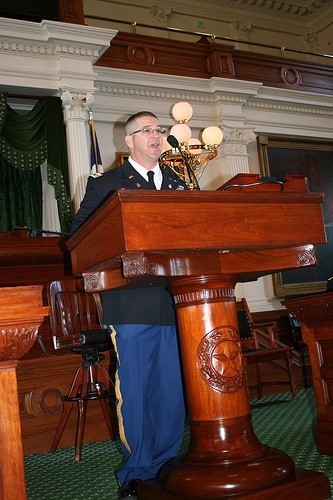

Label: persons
[65,111,194,500]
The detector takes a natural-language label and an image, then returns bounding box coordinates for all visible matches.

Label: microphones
[167,135,200,189]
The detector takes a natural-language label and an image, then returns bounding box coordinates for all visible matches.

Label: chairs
[231,299,298,407]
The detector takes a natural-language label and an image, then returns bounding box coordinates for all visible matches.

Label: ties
[147,171,157,190]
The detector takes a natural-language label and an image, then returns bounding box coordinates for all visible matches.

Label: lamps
[155,102,224,191]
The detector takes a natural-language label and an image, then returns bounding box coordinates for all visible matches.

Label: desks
[281,288,333,458]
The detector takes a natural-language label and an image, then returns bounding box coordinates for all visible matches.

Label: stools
[44,279,122,461]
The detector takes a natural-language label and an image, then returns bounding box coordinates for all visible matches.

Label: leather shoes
[120,478,139,500]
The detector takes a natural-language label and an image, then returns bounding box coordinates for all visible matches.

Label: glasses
[130,128,167,135]
